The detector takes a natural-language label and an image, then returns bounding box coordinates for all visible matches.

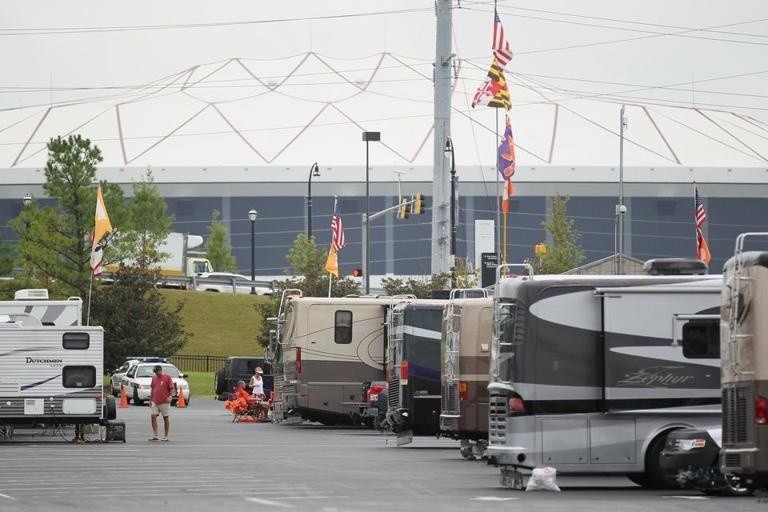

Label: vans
[91,227,216,276]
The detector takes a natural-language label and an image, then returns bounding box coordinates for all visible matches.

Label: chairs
[232,386,270,423]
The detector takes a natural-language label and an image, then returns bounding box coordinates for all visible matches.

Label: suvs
[195,271,281,296]
[214,356,274,401]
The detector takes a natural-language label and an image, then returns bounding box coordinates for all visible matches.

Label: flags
[497,115,515,178]
[324,241,339,279]
[470,8,515,110]
[331,204,346,253]
[94,190,112,251]
[501,177,512,215]
[90,228,103,276]
[693,183,711,265]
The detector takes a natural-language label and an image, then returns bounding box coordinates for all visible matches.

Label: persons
[249,367,266,417]
[236,381,265,418]
[148,366,175,441]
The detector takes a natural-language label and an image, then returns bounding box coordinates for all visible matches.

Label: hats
[153,366,161,372]
[255,367,264,373]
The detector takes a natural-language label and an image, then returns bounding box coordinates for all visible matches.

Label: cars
[110,360,140,396]
[120,363,189,405]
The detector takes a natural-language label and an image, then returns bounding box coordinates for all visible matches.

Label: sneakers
[161,437,168,440]
[148,437,159,440]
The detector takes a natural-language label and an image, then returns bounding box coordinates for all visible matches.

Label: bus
[486,263,756,495]
[719,230,768,483]
[383,298,440,436]
[439,288,492,442]
[273,298,405,424]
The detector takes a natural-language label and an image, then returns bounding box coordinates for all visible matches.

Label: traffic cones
[176,386,186,408]
[119,385,128,407]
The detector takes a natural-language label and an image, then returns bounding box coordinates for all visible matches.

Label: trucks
[0,281,105,449]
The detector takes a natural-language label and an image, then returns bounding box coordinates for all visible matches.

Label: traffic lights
[405,201,410,219]
[420,194,425,214]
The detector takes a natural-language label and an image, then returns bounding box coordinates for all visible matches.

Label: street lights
[22,193,33,279]
[246,209,258,294]
[444,137,459,271]
[305,161,320,238]
